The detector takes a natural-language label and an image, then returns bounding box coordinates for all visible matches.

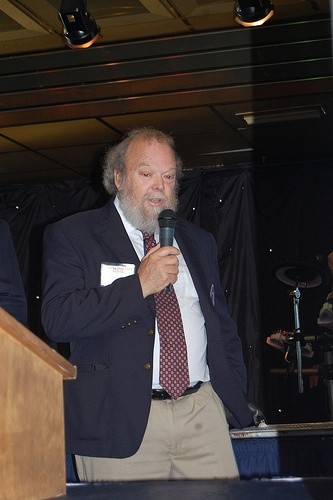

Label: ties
[136,225,190,399]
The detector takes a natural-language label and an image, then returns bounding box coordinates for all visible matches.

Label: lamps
[57,0,103,49]
[233,0,275,28]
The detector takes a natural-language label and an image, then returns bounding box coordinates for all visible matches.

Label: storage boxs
[229,423,333,480]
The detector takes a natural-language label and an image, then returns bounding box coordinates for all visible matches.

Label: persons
[0,220,28,324]
[40,128,253,482]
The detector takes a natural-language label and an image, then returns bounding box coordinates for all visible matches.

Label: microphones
[306,255,323,263]
[158,209,176,293]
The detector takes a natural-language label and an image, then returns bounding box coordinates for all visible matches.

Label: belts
[150,380,204,400]
